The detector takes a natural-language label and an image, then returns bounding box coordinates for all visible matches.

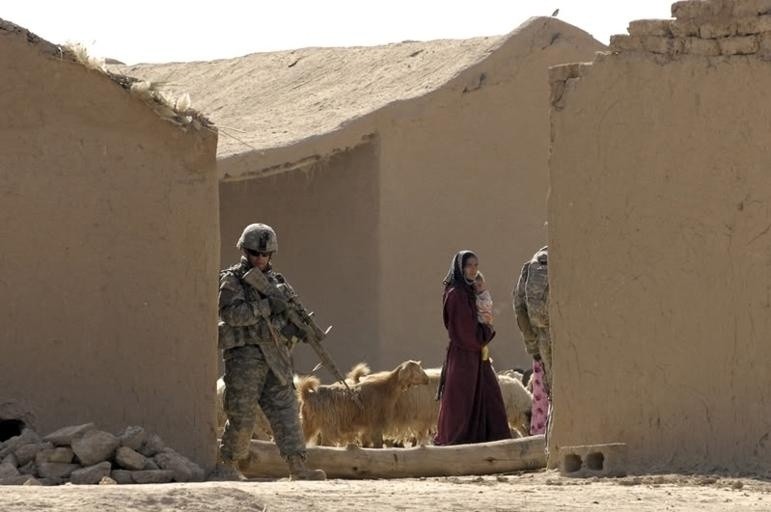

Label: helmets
[236,223,279,255]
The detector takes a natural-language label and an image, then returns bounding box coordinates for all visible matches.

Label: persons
[474,272,495,367]
[215,222,330,481]
[433,249,514,445]
[512,243,552,401]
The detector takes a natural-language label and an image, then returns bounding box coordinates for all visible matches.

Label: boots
[286,454,329,481]
[214,452,250,482]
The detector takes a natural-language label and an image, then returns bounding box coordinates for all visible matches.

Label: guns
[242,265,365,412]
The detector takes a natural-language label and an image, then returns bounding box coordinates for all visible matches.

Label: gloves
[268,297,288,314]
